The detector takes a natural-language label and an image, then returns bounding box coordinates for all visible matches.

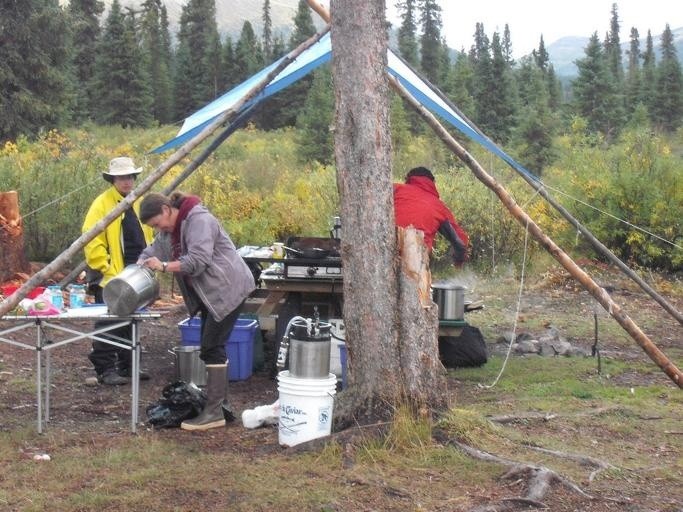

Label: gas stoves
[283,252,341,279]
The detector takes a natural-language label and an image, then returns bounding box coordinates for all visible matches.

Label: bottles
[48,285,86,309]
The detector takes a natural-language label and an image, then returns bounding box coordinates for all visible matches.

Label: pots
[102,263,161,316]
[167,346,208,387]
[281,245,331,259]
[430,285,466,322]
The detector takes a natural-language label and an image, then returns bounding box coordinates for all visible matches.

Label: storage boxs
[179,317,258,381]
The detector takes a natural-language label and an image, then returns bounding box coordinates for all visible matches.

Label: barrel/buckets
[336,344,349,391]
[276,368,336,448]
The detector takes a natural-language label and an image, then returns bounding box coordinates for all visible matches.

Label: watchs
[163,261,168,273]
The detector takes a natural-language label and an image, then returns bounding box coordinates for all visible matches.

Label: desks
[2,306,167,433]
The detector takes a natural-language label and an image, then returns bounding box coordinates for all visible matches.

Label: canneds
[70,285,86,308]
[47,285,62,309]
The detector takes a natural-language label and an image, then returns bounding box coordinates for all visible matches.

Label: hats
[102,156,144,184]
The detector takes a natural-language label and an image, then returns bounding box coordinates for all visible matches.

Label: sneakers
[97,365,150,385]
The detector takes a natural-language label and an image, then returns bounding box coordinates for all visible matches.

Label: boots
[179,359,230,430]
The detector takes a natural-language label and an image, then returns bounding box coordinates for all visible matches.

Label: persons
[136,192,256,432]
[392,166,468,269]
[82,157,156,385]
